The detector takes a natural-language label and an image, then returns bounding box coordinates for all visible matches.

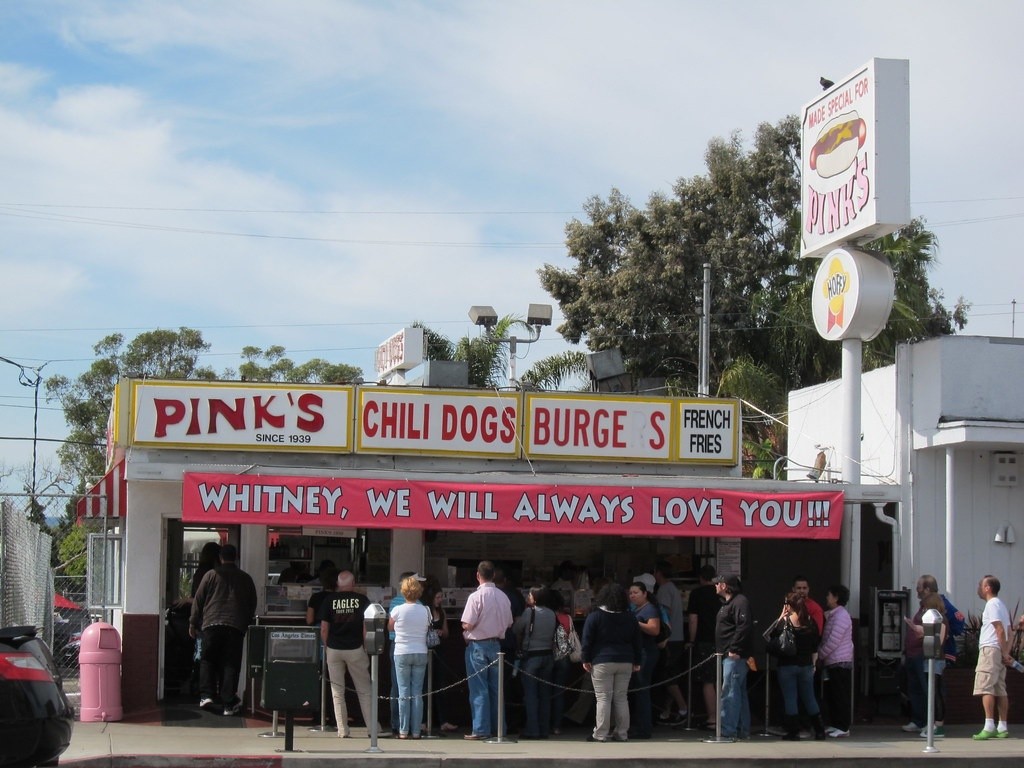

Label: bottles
[1012,661,1024,673]
[269,538,289,557]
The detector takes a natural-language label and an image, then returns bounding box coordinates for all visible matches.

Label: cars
[0,625,74,768]
[60,640,82,668]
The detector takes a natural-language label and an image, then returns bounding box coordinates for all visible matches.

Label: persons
[320,570,382,738]
[912,593,948,737]
[174,542,257,715]
[818,584,853,738]
[460,561,513,740]
[704,573,755,742]
[972,574,1012,739]
[386,576,432,739]
[901,574,939,731]
[771,593,826,742]
[277,560,726,734]
[581,584,642,742]
[776,576,823,736]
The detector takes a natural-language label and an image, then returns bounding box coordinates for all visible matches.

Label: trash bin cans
[76,621,125,722]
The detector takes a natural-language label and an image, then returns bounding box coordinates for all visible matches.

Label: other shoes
[652,713,671,725]
[441,724,458,733]
[223,707,235,716]
[902,722,925,732]
[464,733,488,740]
[200,698,213,708]
[696,720,716,731]
[670,711,694,725]
[920,724,945,738]
[825,727,850,737]
[586,735,606,743]
[973,727,1008,740]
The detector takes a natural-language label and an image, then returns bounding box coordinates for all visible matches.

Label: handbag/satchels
[426,625,440,649]
[568,614,583,664]
[553,624,573,662]
[512,650,527,679]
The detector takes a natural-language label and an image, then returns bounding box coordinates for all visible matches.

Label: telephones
[888,610,896,630]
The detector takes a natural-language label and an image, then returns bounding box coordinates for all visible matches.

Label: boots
[782,713,800,741]
[811,713,825,740]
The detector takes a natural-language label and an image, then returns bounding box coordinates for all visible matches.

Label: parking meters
[363,604,386,752]
[922,609,942,756]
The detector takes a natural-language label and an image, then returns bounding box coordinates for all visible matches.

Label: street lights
[469,305,554,385]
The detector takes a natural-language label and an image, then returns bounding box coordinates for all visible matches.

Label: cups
[297,548,308,558]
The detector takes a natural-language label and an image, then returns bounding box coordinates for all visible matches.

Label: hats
[633,573,656,594]
[712,574,740,586]
